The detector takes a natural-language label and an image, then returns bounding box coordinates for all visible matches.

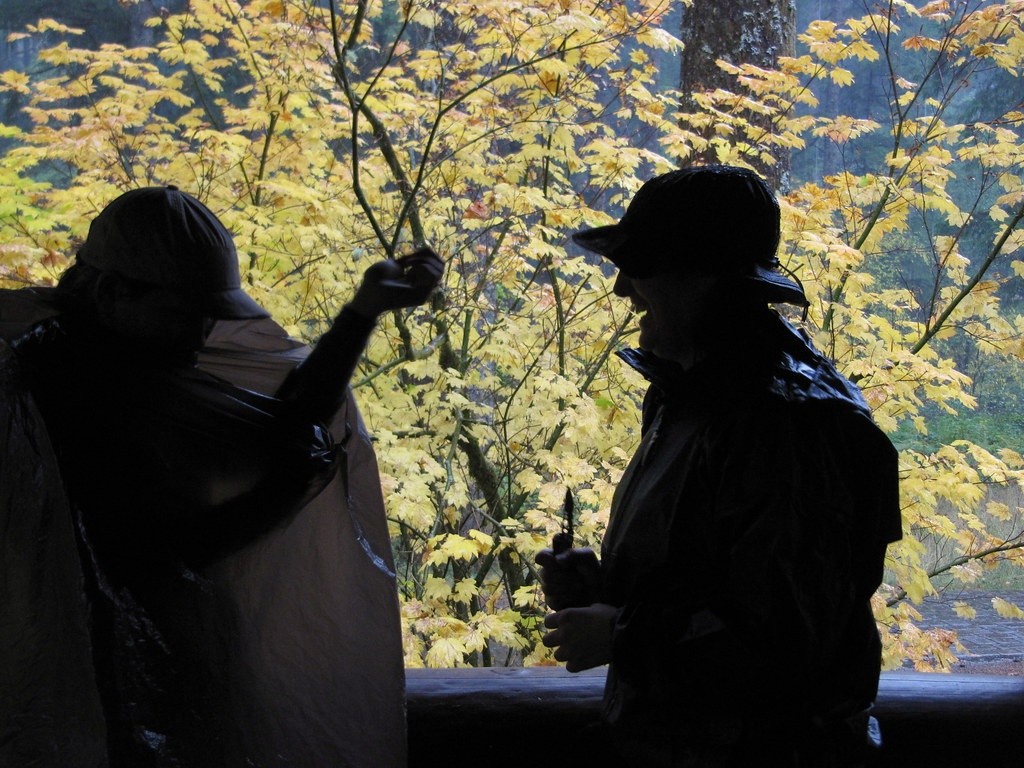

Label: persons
[536,166,905,768]
[0,186,444,768]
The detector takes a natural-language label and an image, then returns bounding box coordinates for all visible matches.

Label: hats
[571,166,808,306]
[78,185,269,320]
